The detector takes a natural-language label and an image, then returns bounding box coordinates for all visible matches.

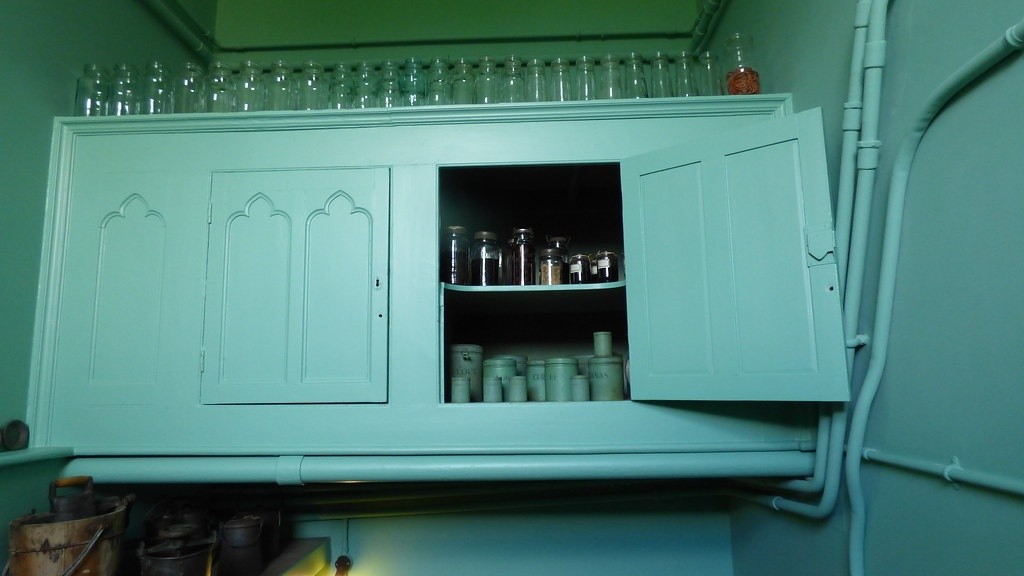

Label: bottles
[441,222,619,286]
[73,63,110,115]
[445,332,626,401]
[107,60,140,115]
[139,30,760,114]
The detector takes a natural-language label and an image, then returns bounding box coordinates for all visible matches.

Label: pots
[218,515,262,547]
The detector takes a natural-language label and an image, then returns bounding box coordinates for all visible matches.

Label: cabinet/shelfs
[26,89,851,487]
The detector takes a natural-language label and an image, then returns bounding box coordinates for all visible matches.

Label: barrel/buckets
[3,491,129,576]
[134,537,219,576]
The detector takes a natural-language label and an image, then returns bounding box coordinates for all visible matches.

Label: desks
[261,537,332,576]
